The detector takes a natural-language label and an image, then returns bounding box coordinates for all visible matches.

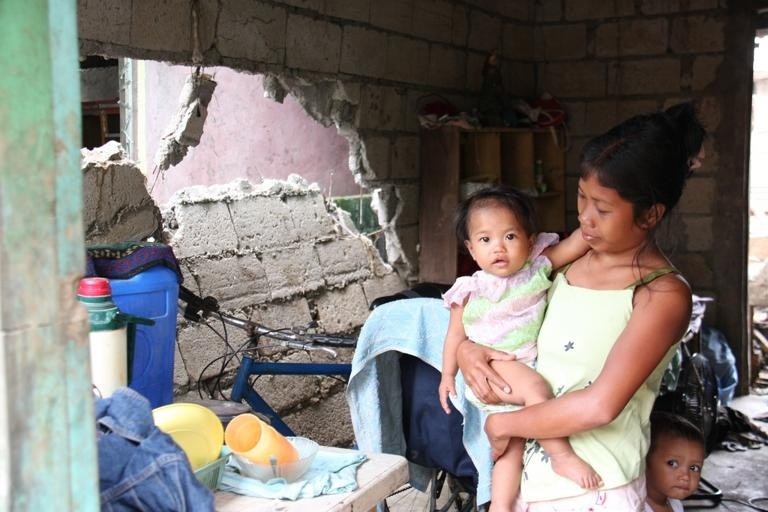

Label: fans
[677,352,723,500]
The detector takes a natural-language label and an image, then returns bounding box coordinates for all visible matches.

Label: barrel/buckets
[104,262,175,406]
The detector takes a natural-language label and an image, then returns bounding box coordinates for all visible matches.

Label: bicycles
[174,283,475,512]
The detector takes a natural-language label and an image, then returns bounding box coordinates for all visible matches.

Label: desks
[212,440,411,512]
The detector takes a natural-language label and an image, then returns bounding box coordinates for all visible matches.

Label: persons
[644,420,705,512]
[437,185,602,512]
[484,101,706,512]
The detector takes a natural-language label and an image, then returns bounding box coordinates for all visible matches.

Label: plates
[232,436,319,484]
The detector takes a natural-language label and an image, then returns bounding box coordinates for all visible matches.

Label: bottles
[224,414,299,464]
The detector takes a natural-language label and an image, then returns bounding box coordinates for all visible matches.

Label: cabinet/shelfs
[420,128,566,289]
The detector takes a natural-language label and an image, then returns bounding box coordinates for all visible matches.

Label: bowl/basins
[151,403,224,471]
